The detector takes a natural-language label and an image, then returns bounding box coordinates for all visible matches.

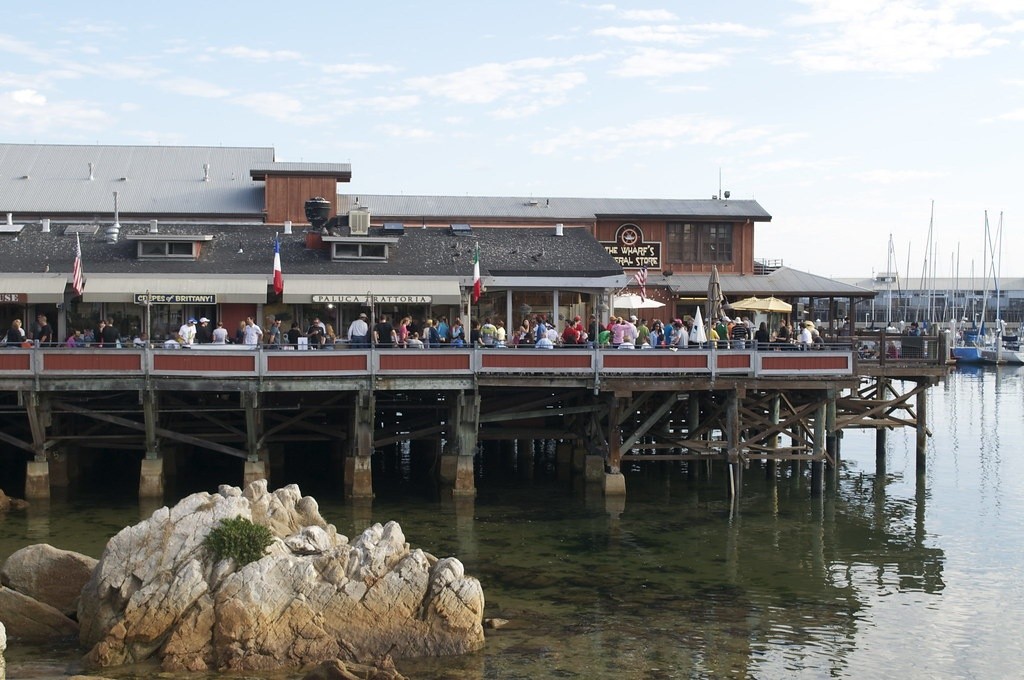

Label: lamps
[712,195,717,199]
[724,191,730,200]
[662,269,673,278]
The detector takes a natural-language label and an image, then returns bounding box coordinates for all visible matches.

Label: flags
[473,248,480,304]
[72,237,84,295]
[635,266,647,302]
[272,238,283,295]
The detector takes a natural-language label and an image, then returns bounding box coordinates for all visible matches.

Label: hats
[199,318,210,323]
[359,314,368,320]
[722,316,732,323]
[630,316,638,320]
[575,316,581,321]
[674,318,683,324]
[589,314,596,320]
[733,317,744,324]
[188,318,197,325]
[610,316,617,321]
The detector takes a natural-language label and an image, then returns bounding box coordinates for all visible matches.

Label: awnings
[282,281,462,304]
[83,279,267,303]
[0,272,68,303]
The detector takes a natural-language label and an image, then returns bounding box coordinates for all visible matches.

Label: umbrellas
[614,293,666,319]
[706,265,725,321]
[689,306,707,345]
[728,297,809,335]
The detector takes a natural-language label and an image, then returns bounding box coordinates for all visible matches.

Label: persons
[236,315,336,350]
[517,315,606,349]
[67,318,181,349]
[347,312,424,348]
[607,316,664,349]
[665,317,751,349]
[843,317,920,360]
[423,317,505,349]
[7,315,52,346]
[176,316,228,344]
[752,319,825,350]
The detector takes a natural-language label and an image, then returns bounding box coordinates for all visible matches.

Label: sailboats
[862,199,1024,364]
